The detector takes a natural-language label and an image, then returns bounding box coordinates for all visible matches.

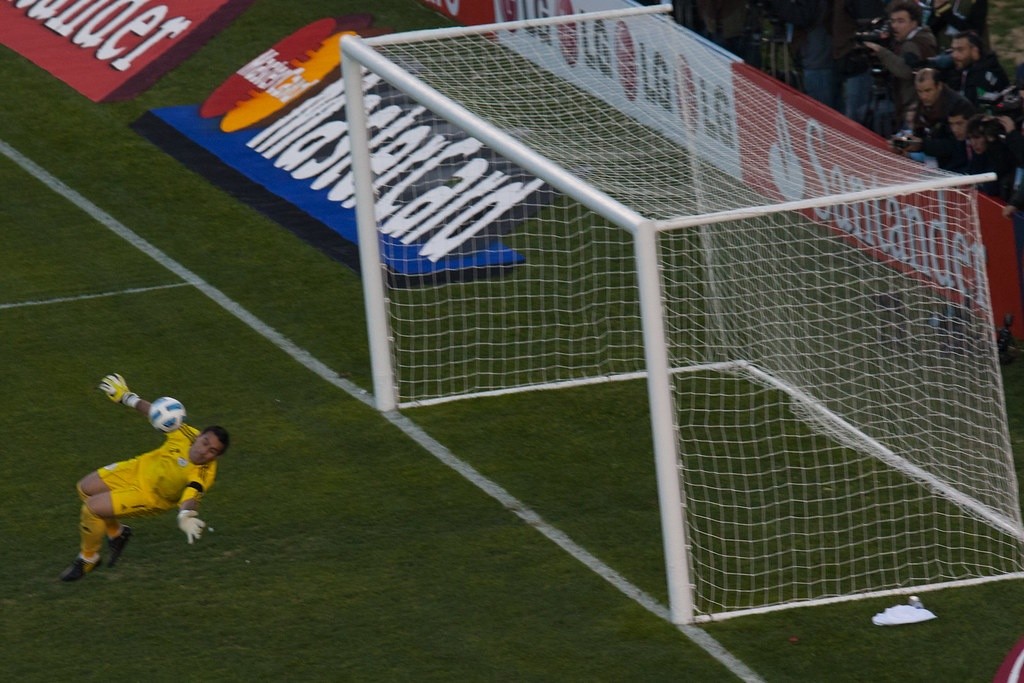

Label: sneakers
[106,525,133,568]
[60,552,102,582]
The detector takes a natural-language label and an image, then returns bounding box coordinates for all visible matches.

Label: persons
[637,0,992,131]
[943,97,976,177]
[883,102,937,167]
[59,372,231,582]
[959,112,1016,203]
[945,29,1010,109]
[886,68,976,169]
[978,61,1024,216]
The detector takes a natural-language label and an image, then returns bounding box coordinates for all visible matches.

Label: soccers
[147,396,187,433]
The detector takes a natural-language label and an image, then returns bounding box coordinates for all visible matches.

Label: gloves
[98,372,130,405]
[176,509,206,545]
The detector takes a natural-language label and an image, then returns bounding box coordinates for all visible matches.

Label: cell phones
[892,134,909,147]
